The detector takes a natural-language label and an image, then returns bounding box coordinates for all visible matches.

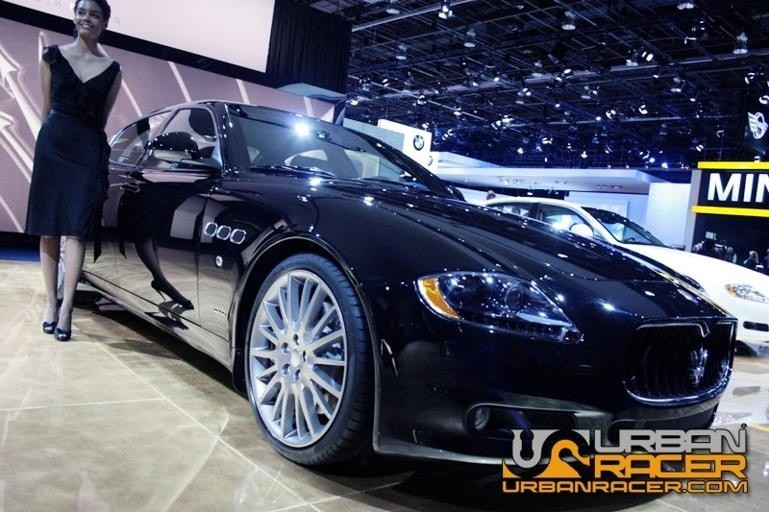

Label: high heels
[42,306,72,342]
[151,280,196,310]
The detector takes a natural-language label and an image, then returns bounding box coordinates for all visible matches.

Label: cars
[53,98,737,468]
[468,198,769,355]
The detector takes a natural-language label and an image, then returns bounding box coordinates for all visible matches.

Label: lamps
[730,36,749,56]
[562,18,577,31]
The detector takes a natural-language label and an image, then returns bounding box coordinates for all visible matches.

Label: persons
[692,237,769,275]
[115,117,194,312]
[25,0,124,342]
